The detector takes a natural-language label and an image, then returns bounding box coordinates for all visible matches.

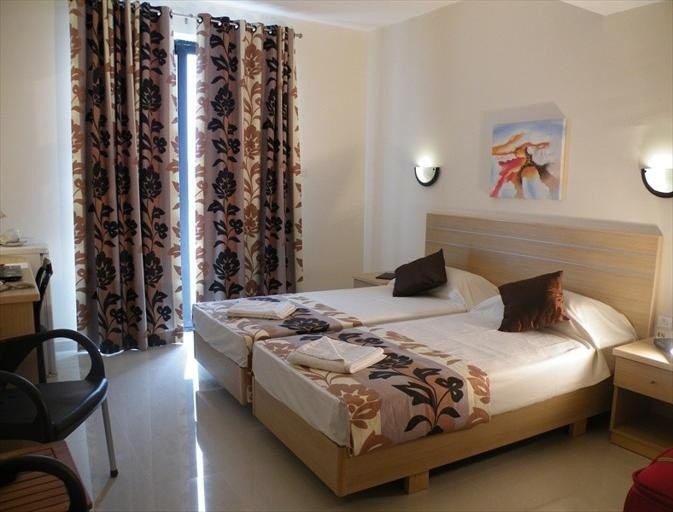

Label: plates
[3,238,29,247]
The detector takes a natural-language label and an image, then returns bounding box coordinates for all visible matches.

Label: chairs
[33,257,53,383]
[0,329,118,478]
[0,454,89,512]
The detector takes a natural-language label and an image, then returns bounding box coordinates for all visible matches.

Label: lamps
[414,167,440,187]
[640,168,673,197]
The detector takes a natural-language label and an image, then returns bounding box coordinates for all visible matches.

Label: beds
[193,205,665,498]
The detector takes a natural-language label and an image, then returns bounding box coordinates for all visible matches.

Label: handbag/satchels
[622,449,673,511]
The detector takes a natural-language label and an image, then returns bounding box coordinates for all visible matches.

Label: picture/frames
[487,118,567,201]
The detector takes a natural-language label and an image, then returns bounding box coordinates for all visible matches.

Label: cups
[7,229,23,243]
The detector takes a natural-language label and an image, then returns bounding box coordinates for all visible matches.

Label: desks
[0,255,41,387]
[0,440,92,512]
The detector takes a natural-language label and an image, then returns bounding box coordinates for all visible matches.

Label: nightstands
[610,337,673,461]
[352,272,388,287]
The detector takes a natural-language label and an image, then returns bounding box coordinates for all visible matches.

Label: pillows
[392,248,447,297]
[499,269,570,331]
[469,287,637,352]
[387,266,499,312]
